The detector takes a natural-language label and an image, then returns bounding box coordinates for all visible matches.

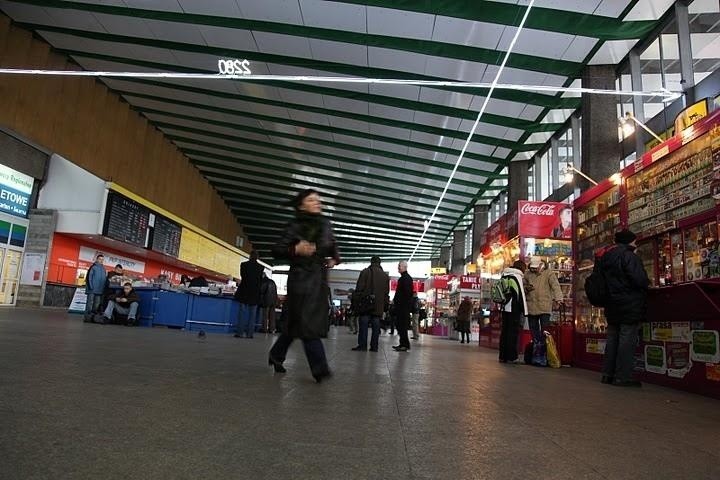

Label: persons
[85,255,107,320]
[522,256,563,345]
[269,190,339,382]
[552,206,574,239]
[392,259,414,351]
[599,227,650,387]
[498,261,527,365]
[351,256,390,352]
[179,249,473,339]
[102,282,140,327]
[106,264,124,285]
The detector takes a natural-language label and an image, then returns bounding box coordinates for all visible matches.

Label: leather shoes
[603,375,641,387]
[352,346,367,351]
[392,345,408,351]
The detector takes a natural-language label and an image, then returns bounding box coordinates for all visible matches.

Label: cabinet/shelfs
[422,202,573,358]
[573,175,622,369]
[622,114,720,393]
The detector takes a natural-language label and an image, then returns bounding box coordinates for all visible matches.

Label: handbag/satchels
[350,293,376,312]
[584,272,607,307]
[524,331,561,368]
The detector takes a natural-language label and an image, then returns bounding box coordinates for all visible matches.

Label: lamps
[617,110,665,146]
[561,163,598,188]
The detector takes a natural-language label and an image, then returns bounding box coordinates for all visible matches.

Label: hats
[530,255,541,268]
[615,229,637,244]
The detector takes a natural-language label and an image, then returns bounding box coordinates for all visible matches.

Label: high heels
[269,357,287,373]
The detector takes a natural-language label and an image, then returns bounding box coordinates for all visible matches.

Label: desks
[109,284,264,334]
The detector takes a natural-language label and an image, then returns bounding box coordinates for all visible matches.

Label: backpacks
[493,276,513,305]
